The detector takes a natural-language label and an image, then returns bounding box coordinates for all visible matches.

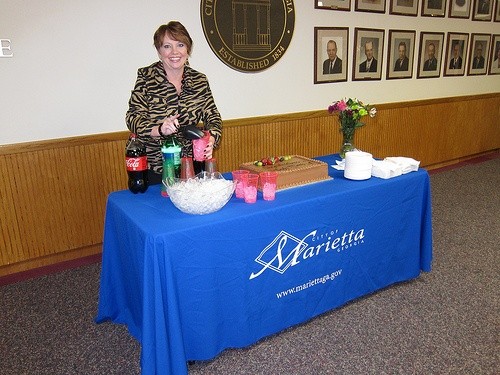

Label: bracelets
[158,123,165,137]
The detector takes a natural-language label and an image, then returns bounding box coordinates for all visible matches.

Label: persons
[423,43,437,71]
[494,49,500,68]
[394,42,409,71]
[126,21,223,186]
[449,43,462,69]
[323,39,342,74]
[472,45,485,69]
[359,40,377,72]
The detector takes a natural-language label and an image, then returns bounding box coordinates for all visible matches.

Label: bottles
[125,133,149,193]
[204,158,219,179]
[161,134,182,197]
[180,158,196,180]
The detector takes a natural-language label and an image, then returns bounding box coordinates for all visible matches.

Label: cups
[232,170,249,198]
[259,172,277,201]
[193,131,210,161]
[240,173,259,204]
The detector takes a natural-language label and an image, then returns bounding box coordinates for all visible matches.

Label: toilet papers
[344,151,372,181]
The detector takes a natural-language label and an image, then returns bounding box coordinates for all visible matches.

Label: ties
[367,61,370,71]
[430,60,431,65]
[455,58,456,66]
[399,58,402,63]
[330,61,332,73]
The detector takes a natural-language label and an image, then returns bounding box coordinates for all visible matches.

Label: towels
[331,156,421,179]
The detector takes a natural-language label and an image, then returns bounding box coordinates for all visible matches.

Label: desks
[94,153,433,374]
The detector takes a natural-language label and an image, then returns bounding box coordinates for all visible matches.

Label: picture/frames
[421,0,447,17]
[313,27,349,84]
[494,0,500,22]
[417,31,445,79]
[448,0,472,19]
[472,0,493,22]
[488,34,500,75]
[351,27,385,81]
[386,30,416,80]
[390,0,419,17]
[355,0,386,14]
[443,31,469,77]
[314,0,351,12]
[466,33,491,76]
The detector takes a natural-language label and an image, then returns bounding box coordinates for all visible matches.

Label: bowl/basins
[162,172,237,213]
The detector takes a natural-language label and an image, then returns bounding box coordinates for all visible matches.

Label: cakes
[239,155,330,192]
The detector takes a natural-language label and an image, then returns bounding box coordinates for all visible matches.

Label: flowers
[327,96,377,142]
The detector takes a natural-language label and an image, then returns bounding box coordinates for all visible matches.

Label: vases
[338,128,355,159]
